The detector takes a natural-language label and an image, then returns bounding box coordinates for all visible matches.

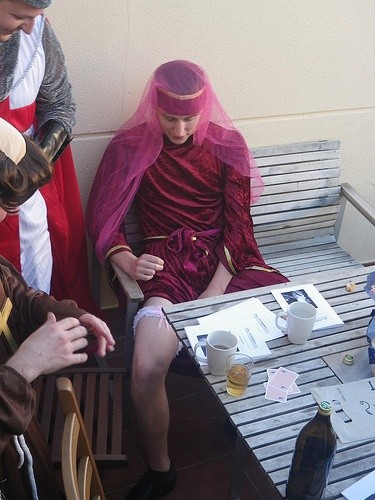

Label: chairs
[27,355,129,500]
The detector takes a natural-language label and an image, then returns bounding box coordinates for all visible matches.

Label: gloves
[33,120,75,164]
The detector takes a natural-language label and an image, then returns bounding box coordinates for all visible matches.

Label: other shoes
[125,459,177,500]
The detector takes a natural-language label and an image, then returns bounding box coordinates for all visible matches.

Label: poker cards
[263,366,302,404]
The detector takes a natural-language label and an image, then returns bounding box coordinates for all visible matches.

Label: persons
[0,1,116,500]
[84,59,291,500]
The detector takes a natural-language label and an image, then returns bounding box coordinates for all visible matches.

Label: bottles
[367,315,375,377]
[286,401,337,499]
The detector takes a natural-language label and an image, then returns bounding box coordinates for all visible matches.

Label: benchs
[90,137,375,387]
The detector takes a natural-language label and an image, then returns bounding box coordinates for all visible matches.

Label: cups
[276,302,317,344]
[225,353,254,397]
[194,331,239,376]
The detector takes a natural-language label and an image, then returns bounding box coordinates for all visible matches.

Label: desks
[161,264,375,500]
